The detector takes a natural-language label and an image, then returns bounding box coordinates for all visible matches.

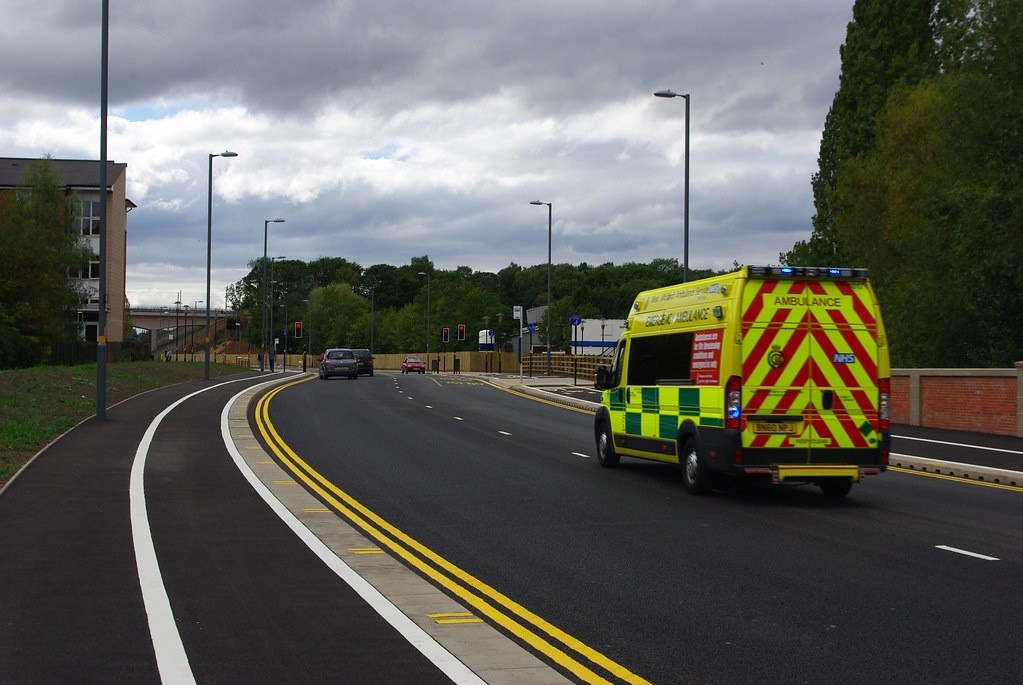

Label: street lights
[279,304,289,366]
[417,271,431,373]
[213,313,218,362]
[260,218,286,375]
[303,299,314,369]
[202,151,240,381]
[496,312,505,374]
[193,300,203,363]
[174,301,182,362]
[183,305,190,362]
[245,315,254,366]
[529,201,553,376]
[652,90,692,279]
[481,316,491,374]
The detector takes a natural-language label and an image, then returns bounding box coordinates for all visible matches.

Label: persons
[257,352,262,368]
[268,345,277,370]
[165,347,172,361]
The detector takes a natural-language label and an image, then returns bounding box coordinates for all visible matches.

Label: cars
[316,348,359,381]
[401,356,426,375]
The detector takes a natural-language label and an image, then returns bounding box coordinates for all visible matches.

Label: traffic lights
[457,324,466,341]
[441,327,450,344]
[294,322,303,339]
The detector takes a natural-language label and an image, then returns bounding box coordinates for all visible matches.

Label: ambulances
[592,264,892,497]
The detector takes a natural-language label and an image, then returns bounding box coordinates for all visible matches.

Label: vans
[351,348,376,377]
